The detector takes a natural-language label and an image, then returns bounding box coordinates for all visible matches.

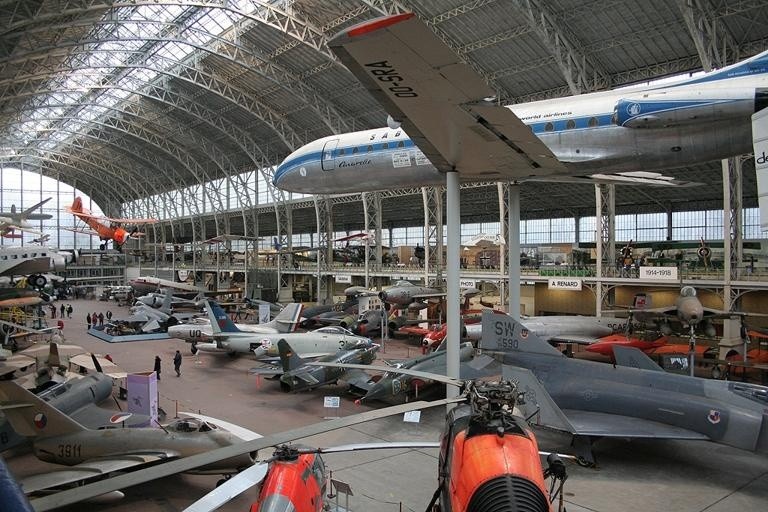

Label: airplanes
[272,13,768,232]
[347,341,482,406]
[0,379,270,500]
[173,411,441,510]
[29,341,579,512]
[1,194,767,465]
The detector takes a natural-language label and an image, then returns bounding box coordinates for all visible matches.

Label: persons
[106,309,112,322]
[67,305,73,318]
[234,305,242,320]
[153,355,161,380]
[99,312,104,326]
[59,304,65,318]
[91,311,97,327]
[50,305,57,319]
[66,303,70,317]
[57,318,64,336]
[174,350,182,377]
[87,313,91,329]
